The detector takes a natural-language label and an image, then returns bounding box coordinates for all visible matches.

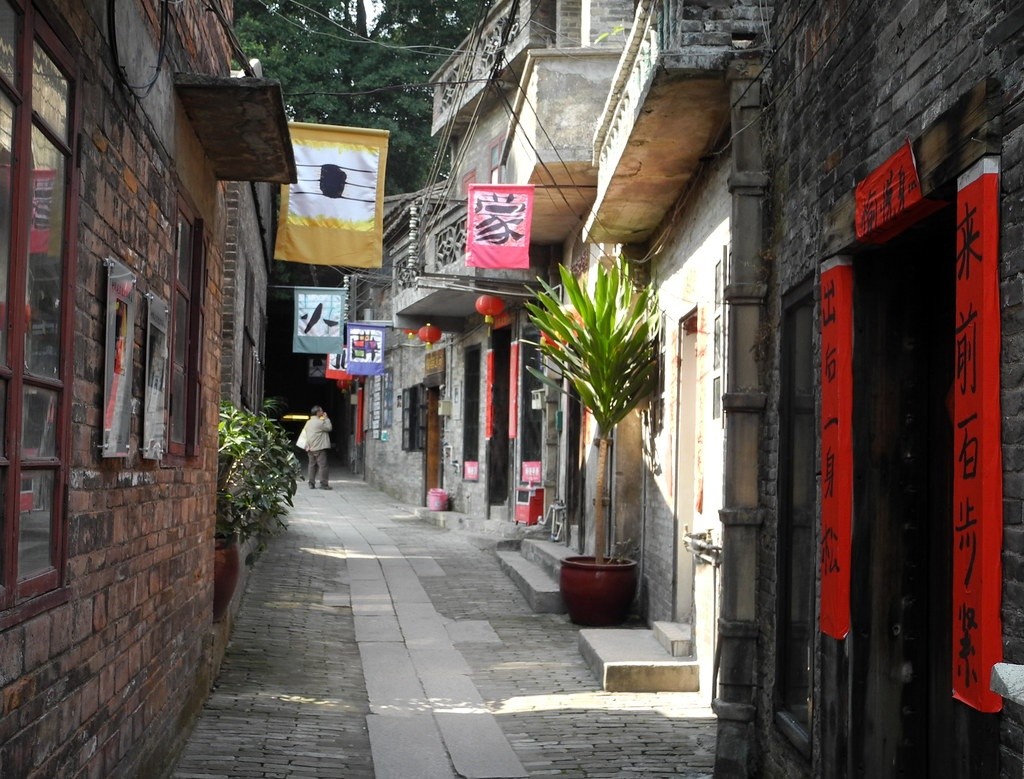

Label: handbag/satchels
[296,429,307,450]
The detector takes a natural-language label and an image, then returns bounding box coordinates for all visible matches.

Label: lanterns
[336,380,349,393]
[418,323,442,349]
[404,329,418,339]
[541,304,585,349]
[475,294,505,324]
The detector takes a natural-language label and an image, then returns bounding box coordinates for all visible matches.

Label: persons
[296,406,333,490]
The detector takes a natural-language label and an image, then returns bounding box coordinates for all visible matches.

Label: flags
[274,121,389,269]
[309,358,326,376]
[347,322,386,375]
[465,183,534,270]
[326,346,356,380]
[293,287,347,354]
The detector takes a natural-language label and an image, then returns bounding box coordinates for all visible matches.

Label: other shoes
[319,485,332,490]
[309,485,315,488]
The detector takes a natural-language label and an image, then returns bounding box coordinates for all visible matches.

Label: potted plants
[524,250,665,628]
[211,397,304,623]
